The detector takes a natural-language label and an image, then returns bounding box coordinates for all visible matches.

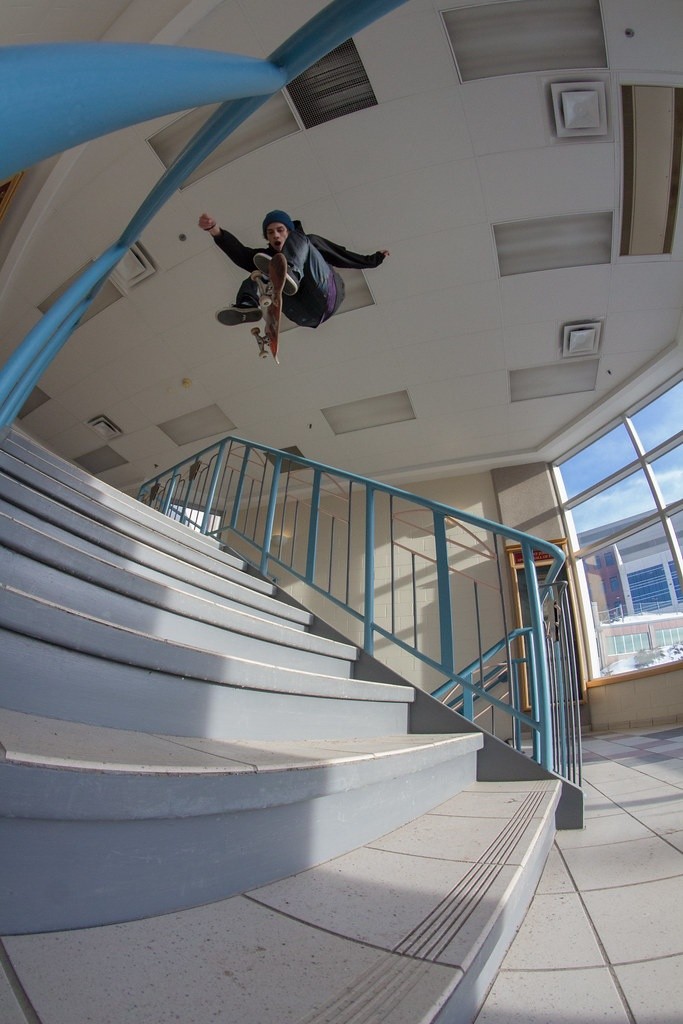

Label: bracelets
[204,223,216,230]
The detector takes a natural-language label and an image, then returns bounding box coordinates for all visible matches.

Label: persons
[198,210,389,328]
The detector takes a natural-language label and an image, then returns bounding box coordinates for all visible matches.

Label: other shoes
[216,303,263,326]
[254,253,299,297]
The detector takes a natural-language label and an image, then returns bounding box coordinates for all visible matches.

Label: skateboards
[248,253,287,367]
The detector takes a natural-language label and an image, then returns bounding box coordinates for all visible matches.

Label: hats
[262,210,294,241]
